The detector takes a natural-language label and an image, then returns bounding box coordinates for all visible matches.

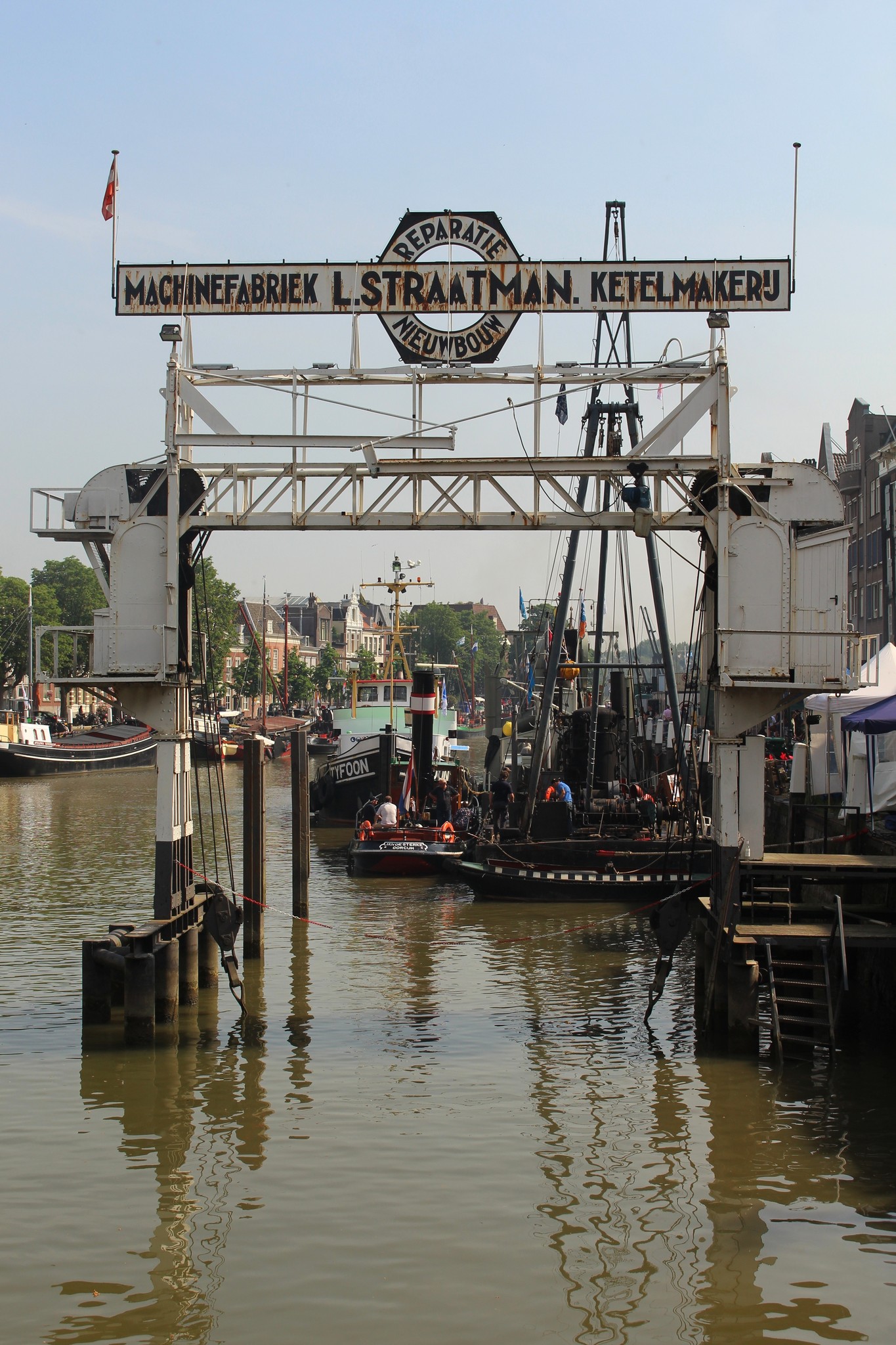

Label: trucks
[268,702,306,718]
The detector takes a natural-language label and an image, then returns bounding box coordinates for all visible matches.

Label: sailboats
[222,573,317,744]
[457,397,719,907]
[1,585,160,773]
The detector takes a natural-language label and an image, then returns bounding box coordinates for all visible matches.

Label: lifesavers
[643,793,655,805]
[545,786,558,802]
[439,821,456,843]
[461,716,465,722]
[358,820,374,841]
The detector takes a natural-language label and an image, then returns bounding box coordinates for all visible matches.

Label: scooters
[76,712,120,725]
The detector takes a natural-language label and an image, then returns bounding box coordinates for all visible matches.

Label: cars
[31,710,67,727]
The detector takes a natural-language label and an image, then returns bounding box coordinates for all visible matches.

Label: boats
[305,733,340,754]
[188,695,222,760]
[456,697,487,738]
[349,666,483,875]
[310,678,459,818]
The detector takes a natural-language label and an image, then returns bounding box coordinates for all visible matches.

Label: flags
[22,683,31,709]
[440,586,607,717]
[326,680,331,690]
[342,682,346,695]
[392,660,396,665]
[331,666,337,677]
[101,158,119,221]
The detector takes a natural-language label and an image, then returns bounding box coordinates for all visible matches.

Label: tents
[790,642,896,819]
[841,694,896,836]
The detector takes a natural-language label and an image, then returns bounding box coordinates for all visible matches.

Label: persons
[79,705,83,715]
[640,704,689,737]
[260,704,263,717]
[756,708,807,742]
[266,704,269,716]
[361,767,573,841]
